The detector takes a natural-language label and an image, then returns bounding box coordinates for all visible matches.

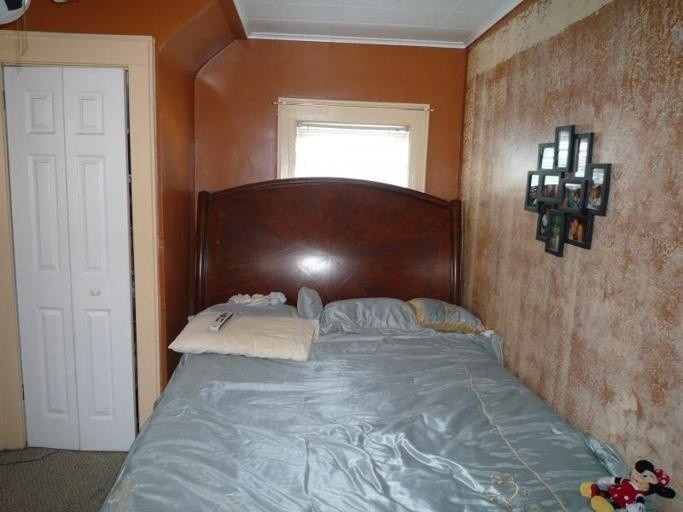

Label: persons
[529,181,601,243]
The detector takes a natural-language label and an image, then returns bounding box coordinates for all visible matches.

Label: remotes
[209,311,234,335]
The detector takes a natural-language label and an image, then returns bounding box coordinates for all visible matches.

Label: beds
[96,176,659,512]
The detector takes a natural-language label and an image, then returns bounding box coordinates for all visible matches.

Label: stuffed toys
[578,459,675,512]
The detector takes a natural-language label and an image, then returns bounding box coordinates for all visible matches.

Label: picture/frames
[524,123,612,257]
